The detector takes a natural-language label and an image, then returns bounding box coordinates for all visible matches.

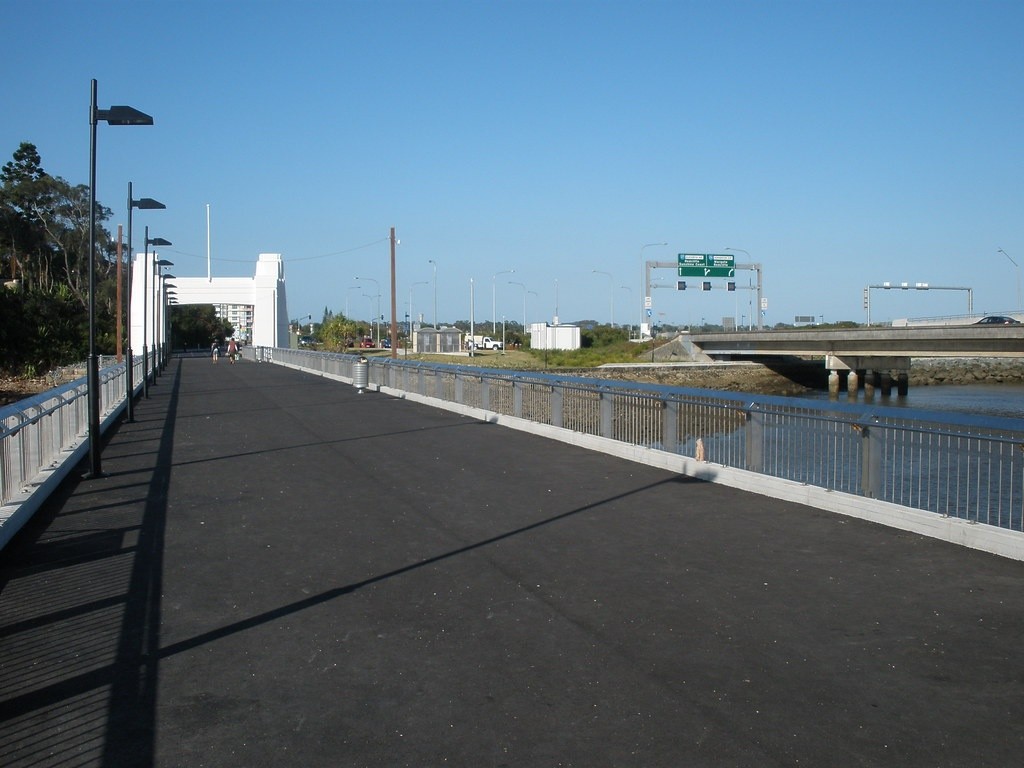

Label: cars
[973,316,1020,325]
[381,339,391,349]
[298,336,317,345]
[360,339,375,348]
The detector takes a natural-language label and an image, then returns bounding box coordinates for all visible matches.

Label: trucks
[464,336,503,350]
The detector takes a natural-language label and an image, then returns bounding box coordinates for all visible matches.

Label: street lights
[152,260,174,385]
[820,315,823,324]
[85,105,155,478]
[741,316,746,326]
[620,285,632,330]
[144,238,173,397]
[347,287,360,321]
[362,294,382,338]
[354,277,380,347]
[162,273,179,373]
[640,243,667,320]
[127,198,166,421]
[528,291,538,323]
[593,271,613,328]
[724,247,752,331]
[493,270,516,334]
[509,281,526,334]
[410,281,428,341]
[429,261,436,329]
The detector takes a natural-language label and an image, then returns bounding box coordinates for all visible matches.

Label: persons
[183,341,188,353]
[212,339,222,365]
[227,339,238,363]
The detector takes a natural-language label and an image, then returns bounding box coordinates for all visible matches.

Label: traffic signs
[677,253,734,277]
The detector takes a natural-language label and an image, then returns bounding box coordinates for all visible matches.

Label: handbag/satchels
[235,353,239,360]
